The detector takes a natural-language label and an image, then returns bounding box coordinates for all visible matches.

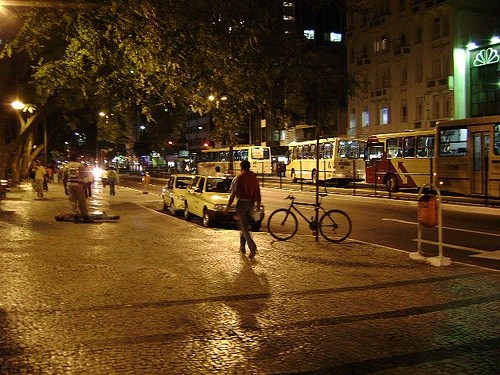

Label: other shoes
[239,248,246,254]
[81,218,93,222]
[74,217,79,223]
[249,248,256,258]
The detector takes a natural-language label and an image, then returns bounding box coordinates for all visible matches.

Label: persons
[275,161,286,177]
[142,171,151,195]
[223,161,261,258]
[213,166,224,187]
[29,154,120,224]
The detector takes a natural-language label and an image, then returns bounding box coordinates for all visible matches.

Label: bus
[285,137,366,185]
[364,130,437,191]
[195,146,272,178]
[433,116,500,198]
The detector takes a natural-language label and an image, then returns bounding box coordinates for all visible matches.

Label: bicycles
[267,193,353,244]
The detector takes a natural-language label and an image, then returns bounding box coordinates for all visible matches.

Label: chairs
[457,148,467,154]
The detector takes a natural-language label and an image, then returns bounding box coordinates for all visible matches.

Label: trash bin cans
[142,175,149,183]
[417,195,438,228]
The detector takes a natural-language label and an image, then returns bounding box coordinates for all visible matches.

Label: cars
[184,176,265,227]
[161,173,194,215]
